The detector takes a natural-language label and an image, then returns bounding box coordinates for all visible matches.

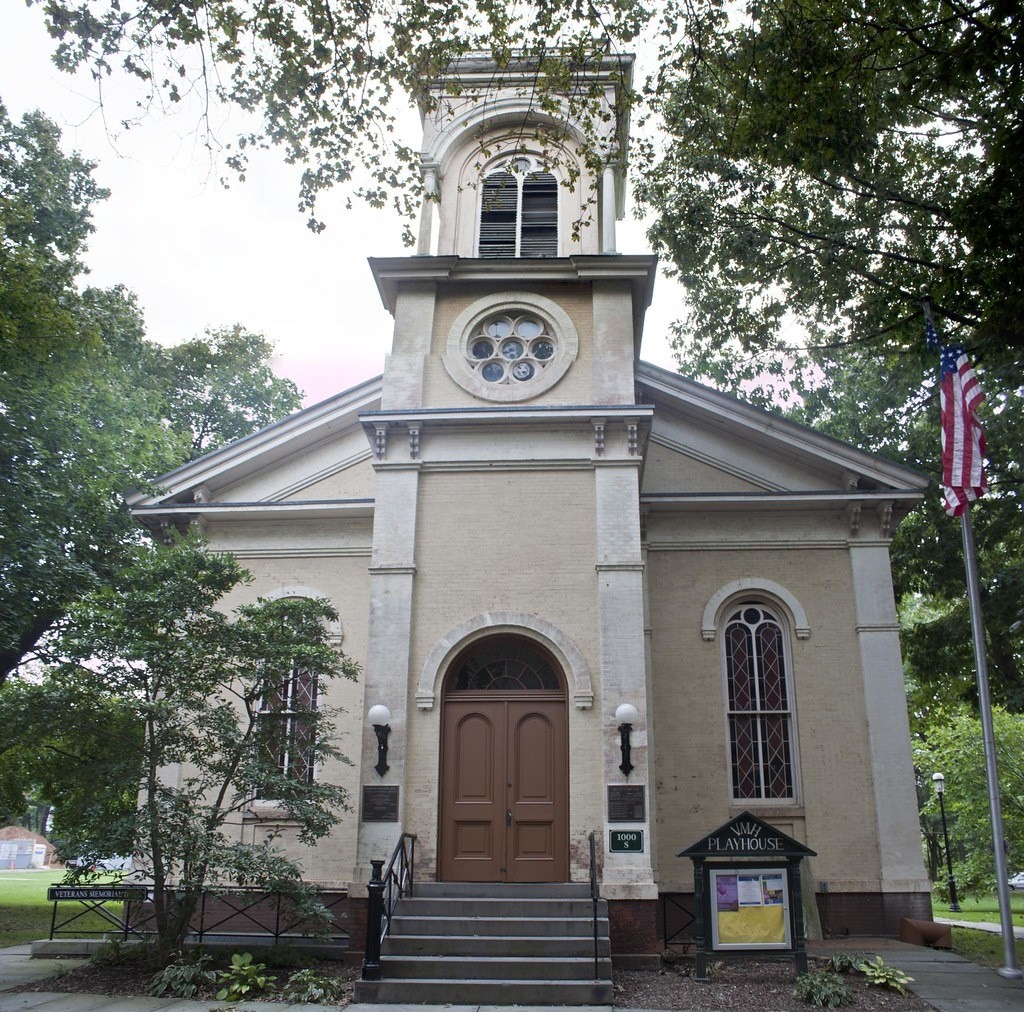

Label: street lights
[931,772,963,912]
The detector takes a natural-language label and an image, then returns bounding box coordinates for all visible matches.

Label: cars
[1007,871,1024,891]
[65,848,132,875]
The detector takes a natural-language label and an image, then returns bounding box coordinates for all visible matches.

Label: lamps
[615,703,638,777]
[368,705,392,778]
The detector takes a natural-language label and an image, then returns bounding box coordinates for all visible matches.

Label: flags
[926,317,984,522]
